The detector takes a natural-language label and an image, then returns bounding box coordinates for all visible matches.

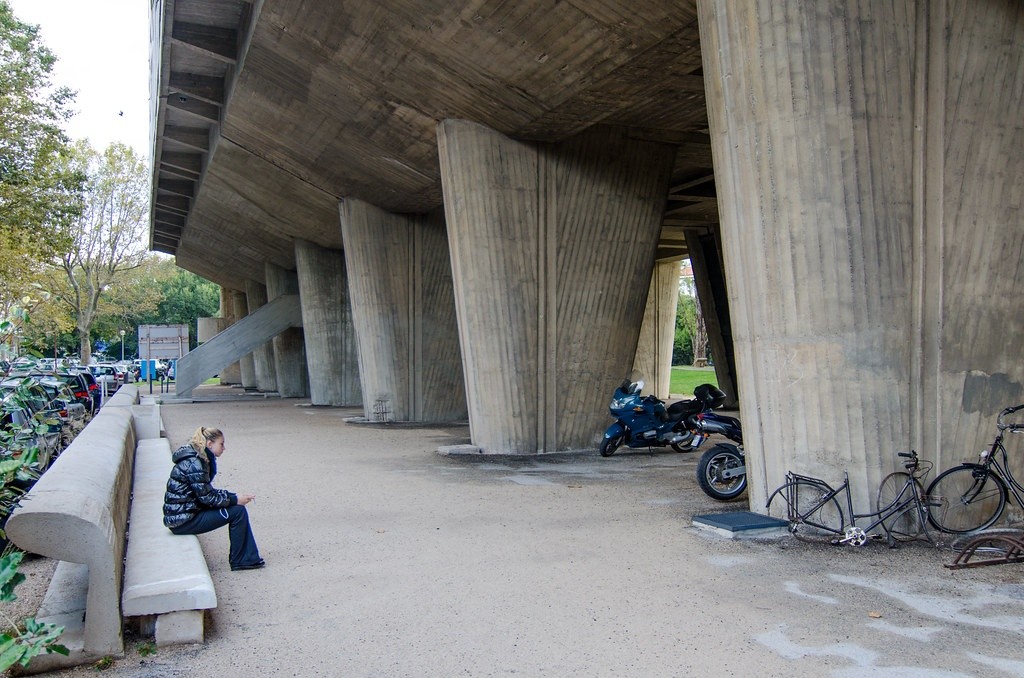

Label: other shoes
[231,557,265,570]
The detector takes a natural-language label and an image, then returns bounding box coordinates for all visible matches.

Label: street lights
[120,329,126,364]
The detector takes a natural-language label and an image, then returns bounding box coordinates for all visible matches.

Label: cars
[0,355,174,555]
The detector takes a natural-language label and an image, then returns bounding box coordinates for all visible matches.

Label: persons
[163,427,265,571]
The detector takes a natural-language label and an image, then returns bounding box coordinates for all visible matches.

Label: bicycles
[925,404,1024,570]
[764,450,948,554]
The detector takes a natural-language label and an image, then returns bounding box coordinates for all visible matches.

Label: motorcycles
[599,379,727,458]
[691,411,748,501]
